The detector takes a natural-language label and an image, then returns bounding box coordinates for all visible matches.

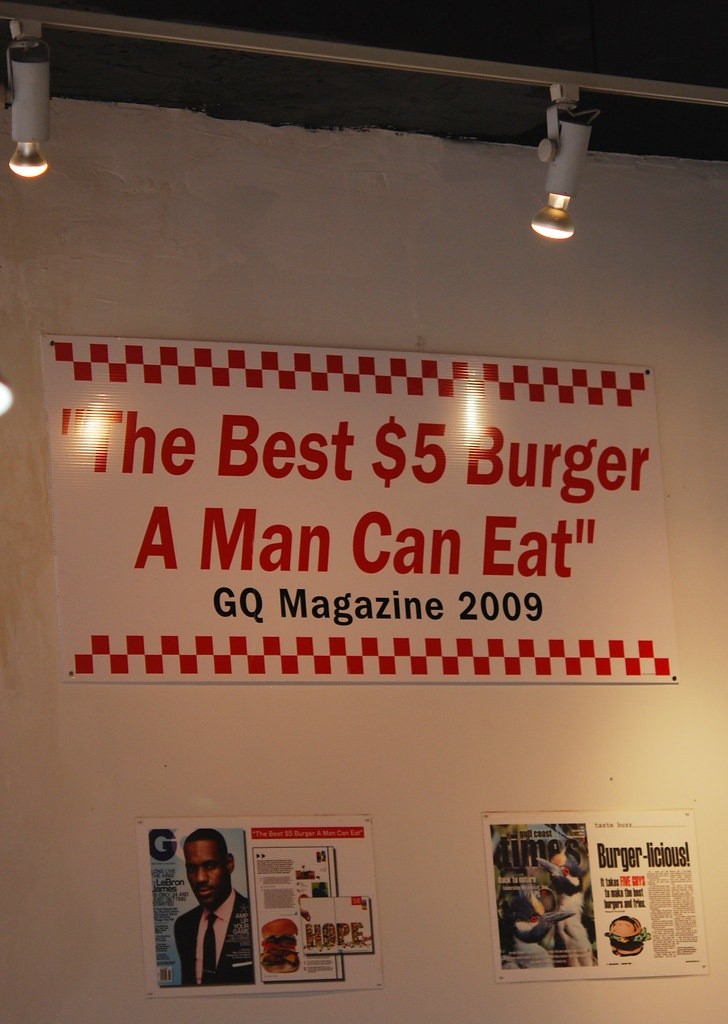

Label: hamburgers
[260,918,300,974]
[602,916,651,959]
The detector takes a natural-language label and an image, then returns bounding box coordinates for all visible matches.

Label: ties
[201,912,219,985]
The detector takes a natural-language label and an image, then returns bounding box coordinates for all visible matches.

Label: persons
[174,828,253,986]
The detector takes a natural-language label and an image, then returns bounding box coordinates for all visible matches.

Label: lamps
[530,121,592,239]
[7,40,51,177]
